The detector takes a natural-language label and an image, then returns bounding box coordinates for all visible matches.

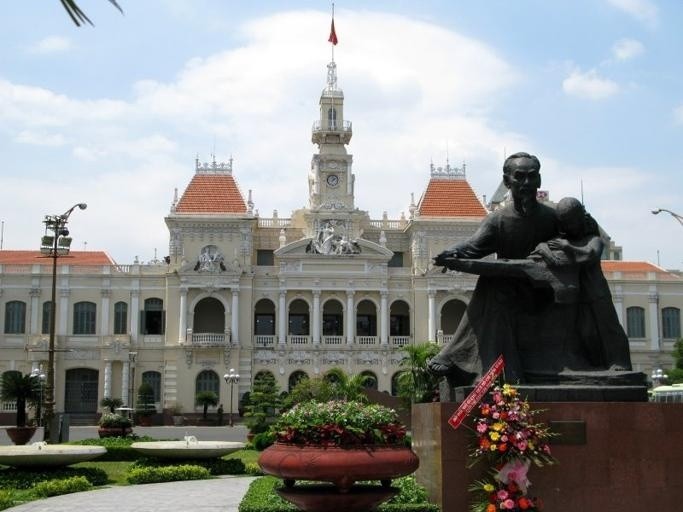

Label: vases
[258,441,417,491]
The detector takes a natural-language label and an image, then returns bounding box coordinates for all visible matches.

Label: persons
[217,404,223,425]
[536,198,632,376]
[318,223,335,254]
[431,151,556,380]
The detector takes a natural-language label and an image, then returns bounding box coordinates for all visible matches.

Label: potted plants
[96,384,156,438]
[0,370,44,444]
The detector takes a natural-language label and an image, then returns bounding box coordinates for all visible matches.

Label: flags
[328,18,338,46]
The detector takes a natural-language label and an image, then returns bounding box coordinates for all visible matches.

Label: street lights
[223,367,240,427]
[34,203,86,439]
[651,369,668,389]
[29,368,45,427]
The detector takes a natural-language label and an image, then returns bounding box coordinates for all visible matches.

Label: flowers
[326,174,338,186]
[460,367,562,512]
[267,398,412,448]
